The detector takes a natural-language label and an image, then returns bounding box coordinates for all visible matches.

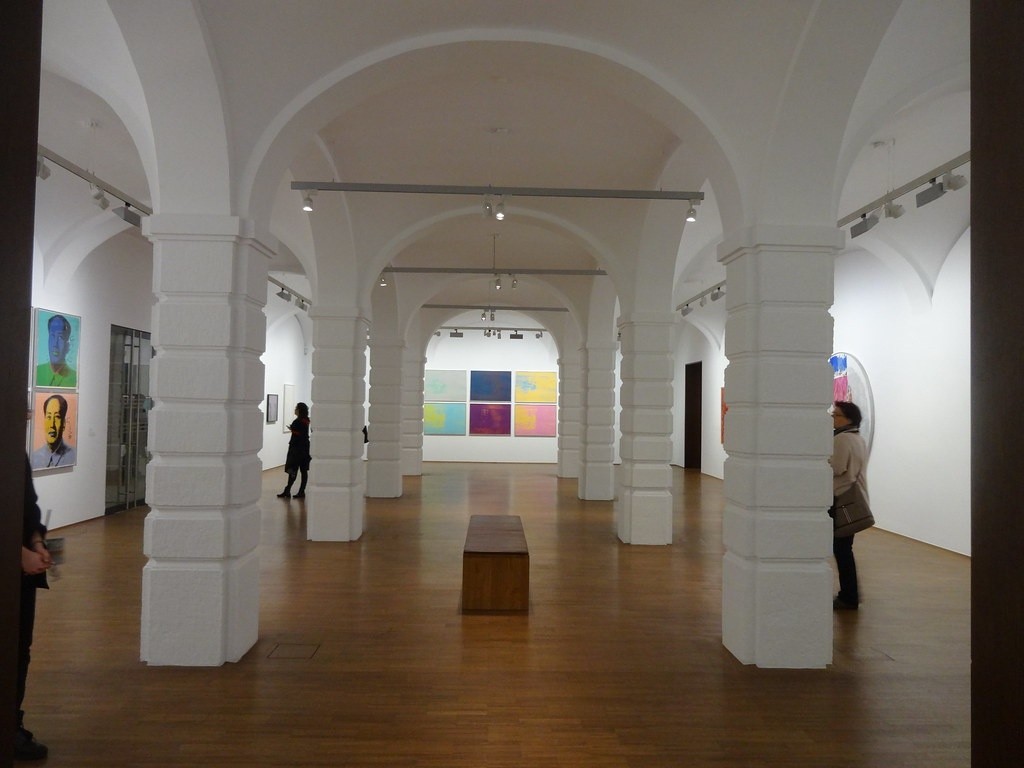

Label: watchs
[32,538,49,549]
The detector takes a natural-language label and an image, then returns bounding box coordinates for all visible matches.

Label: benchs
[456,512,533,619]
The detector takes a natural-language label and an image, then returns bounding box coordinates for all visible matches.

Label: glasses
[831,412,846,418]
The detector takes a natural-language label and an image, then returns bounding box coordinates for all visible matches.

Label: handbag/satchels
[833,483,875,538]
[362,426,369,443]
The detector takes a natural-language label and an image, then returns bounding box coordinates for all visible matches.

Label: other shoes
[833,598,858,610]
[293,490,305,498]
[277,490,291,498]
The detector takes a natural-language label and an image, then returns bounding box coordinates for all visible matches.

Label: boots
[14,710,48,760]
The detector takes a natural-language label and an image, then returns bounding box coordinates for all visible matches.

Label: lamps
[536,330,543,339]
[479,192,513,222]
[36,156,52,180]
[493,273,504,290]
[509,329,524,339]
[111,203,141,227]
[302,189,320,213]
[685,198,702,223]
[847,167,966,240]
[710,286,726,301]
[509,273,520,291]
[680,302,694,317]
[484,329,501,339]
[379,272,388,287]
[275,288,309,311]
[480,308,489,321]
[90,184,110,208]
[433,329,443,336]
[450,329,464,338]
[699,295,708,306]
[490,308,496,321]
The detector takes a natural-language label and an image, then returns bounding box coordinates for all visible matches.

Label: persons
[11,450,55,759]
[828,398,865,614]
[277,402,313,498]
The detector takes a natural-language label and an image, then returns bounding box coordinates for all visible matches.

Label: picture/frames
[424,370,558,437]
[266,393,279,423]
[282,384,296,434]
[33,307,81,390]
[29,389,79,471]
